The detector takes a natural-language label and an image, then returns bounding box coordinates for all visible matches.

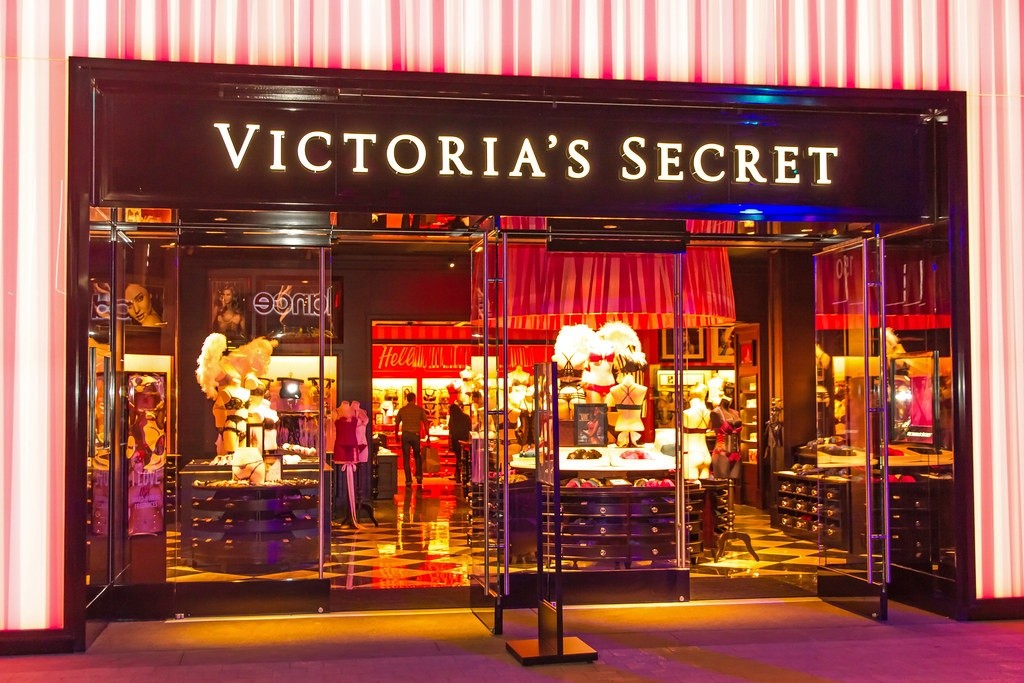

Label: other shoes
[456,479,462,483]
[417,479,422,484]
[406,483,411,487]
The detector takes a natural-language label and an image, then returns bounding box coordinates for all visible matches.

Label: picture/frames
[254,273,345,345]
[573,404,609,448]
[656,328,706,363]
[208,276,252,344]
[711,327,736,363]
[738,339,755,367]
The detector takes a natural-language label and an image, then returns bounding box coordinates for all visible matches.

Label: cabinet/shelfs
[465,468,734,567]
[733,375,759,464]
[179,458,335,577]
[816,366,835,441]
[773,475,957,561]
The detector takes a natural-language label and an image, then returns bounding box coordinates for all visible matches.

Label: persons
[581,334,615,405]
[834,401,846,434]
[579,406,603,444]
[606,375,647,430]
[690,373,733,408]
[213,356,279,454]
[91,281,111,318]
[277,287,333,332]
[815,343,830,408]
[332,401,369,464]
[214,286,248,339]
[477,408,496,468]
[885,345,912,439]
[231,447,266,485]
[557,375,582,420]
[460,366,491,408]
[447,399,472,479]
[123,281,163,329]
[711,400,743,480]
[491,367,547,462]
[941,380,952,449]
[684,398,710,480]
[395,392,429,484]
